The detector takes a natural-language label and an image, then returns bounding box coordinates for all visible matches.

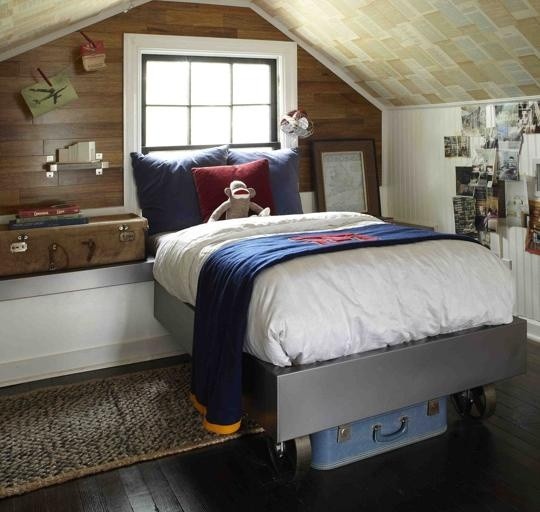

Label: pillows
[128,144,304,232]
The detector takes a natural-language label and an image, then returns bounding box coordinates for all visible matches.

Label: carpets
[1,361,246,497]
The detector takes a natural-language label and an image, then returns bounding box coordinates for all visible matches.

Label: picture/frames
[311,137,381,218]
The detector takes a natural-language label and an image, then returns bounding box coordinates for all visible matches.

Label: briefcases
[310,396,448,471]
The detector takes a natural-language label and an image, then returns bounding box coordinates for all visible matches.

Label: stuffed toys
[206,178,272,222]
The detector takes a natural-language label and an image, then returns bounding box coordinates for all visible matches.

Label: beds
[144,212,528,493]
